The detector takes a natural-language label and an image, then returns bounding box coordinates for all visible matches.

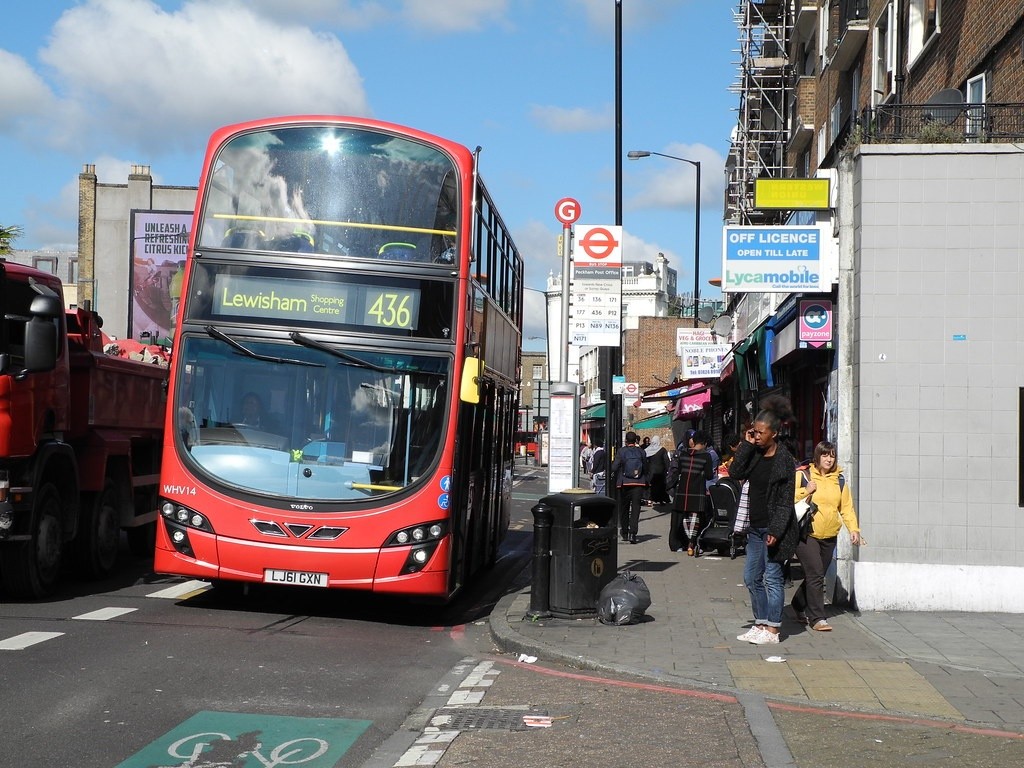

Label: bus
[154,114,523,617]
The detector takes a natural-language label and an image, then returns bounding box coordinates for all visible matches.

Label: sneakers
[813,620,832,631]
[749,627,780,644]
[791,605,809,624]
[736,625,766,641]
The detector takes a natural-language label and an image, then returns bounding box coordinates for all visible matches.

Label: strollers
[695,476,748,559]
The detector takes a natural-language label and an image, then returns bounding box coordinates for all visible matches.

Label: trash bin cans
[540,489,618,620]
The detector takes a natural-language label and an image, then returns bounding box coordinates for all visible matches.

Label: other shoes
[620,529,628,539]
[677,547,683,552]
[687,549,694,556]
[629,534,637,544]
[693,548,703,553]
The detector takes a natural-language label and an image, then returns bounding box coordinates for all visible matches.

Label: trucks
[0,255,167,604]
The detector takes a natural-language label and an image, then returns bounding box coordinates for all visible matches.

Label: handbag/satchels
[794,469,818,545]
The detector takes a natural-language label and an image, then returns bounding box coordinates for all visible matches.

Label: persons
[580,396,862,644]
[234,393,279,434]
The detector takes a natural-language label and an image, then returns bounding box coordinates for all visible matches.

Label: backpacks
[621,446,643,479]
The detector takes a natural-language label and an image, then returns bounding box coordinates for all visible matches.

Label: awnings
[634,378,712,421]
[631,409,674,429]
[716,323,772,394]
[580,404,606,418]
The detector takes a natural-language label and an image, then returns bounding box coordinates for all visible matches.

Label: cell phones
[751,432,754,438]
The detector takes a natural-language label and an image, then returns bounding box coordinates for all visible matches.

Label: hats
[594,439,603,446]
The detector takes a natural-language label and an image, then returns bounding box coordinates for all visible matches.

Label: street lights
[627,151,701,329]
